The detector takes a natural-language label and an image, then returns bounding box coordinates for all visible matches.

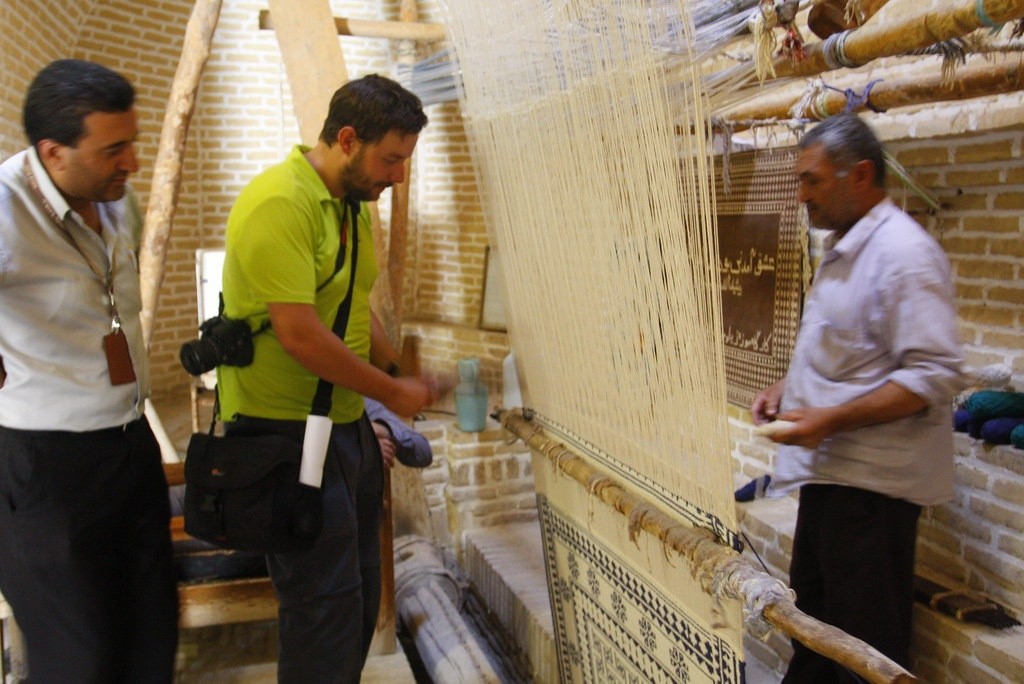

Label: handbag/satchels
[183,432,323,551]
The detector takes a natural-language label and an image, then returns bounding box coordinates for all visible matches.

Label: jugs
[454,358,488,431]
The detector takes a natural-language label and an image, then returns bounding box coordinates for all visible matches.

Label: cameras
[179,318,254,375]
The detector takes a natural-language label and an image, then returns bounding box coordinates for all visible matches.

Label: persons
[749,114,963,684]
[0,59,181,684]
[213,73,436,684]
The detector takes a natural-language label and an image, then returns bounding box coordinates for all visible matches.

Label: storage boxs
[163,463,282,673]
